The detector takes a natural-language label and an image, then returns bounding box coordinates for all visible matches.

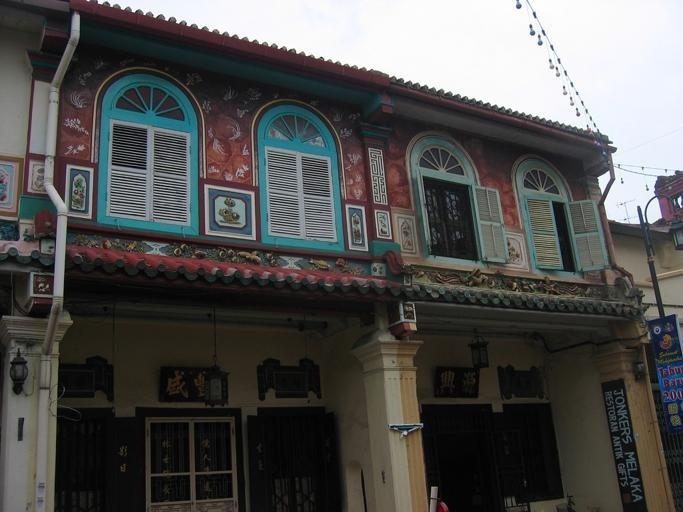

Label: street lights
[636,195,682,318]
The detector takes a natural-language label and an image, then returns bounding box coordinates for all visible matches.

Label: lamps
[468,328,490,369]
[8,346,27,396]
[201,307,231,408]
